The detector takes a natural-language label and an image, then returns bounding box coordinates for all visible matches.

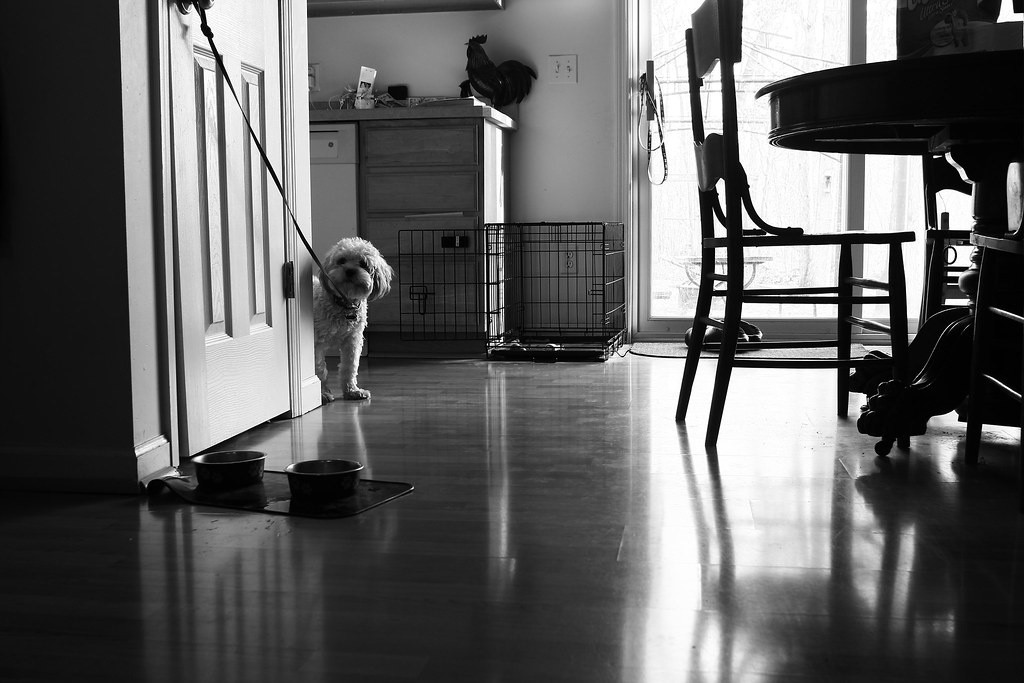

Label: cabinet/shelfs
[310,107,519,360]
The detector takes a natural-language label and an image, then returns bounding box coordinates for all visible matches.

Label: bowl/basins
[190,450,268,488]
[285,459,364,496]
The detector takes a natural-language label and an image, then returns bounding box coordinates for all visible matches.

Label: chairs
[965,216,1024,455]
[920,145,975,312]
[675,0,916,449]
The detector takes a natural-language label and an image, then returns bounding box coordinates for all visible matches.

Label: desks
[751,55,1023,454]
[681,253,773,315]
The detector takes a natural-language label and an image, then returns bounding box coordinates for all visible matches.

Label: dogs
[312,237,395,405]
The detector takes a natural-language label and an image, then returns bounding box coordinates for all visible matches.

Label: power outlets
[547,54,579,85]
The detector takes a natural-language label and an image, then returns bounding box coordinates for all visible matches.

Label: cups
[356,95,377,110]
